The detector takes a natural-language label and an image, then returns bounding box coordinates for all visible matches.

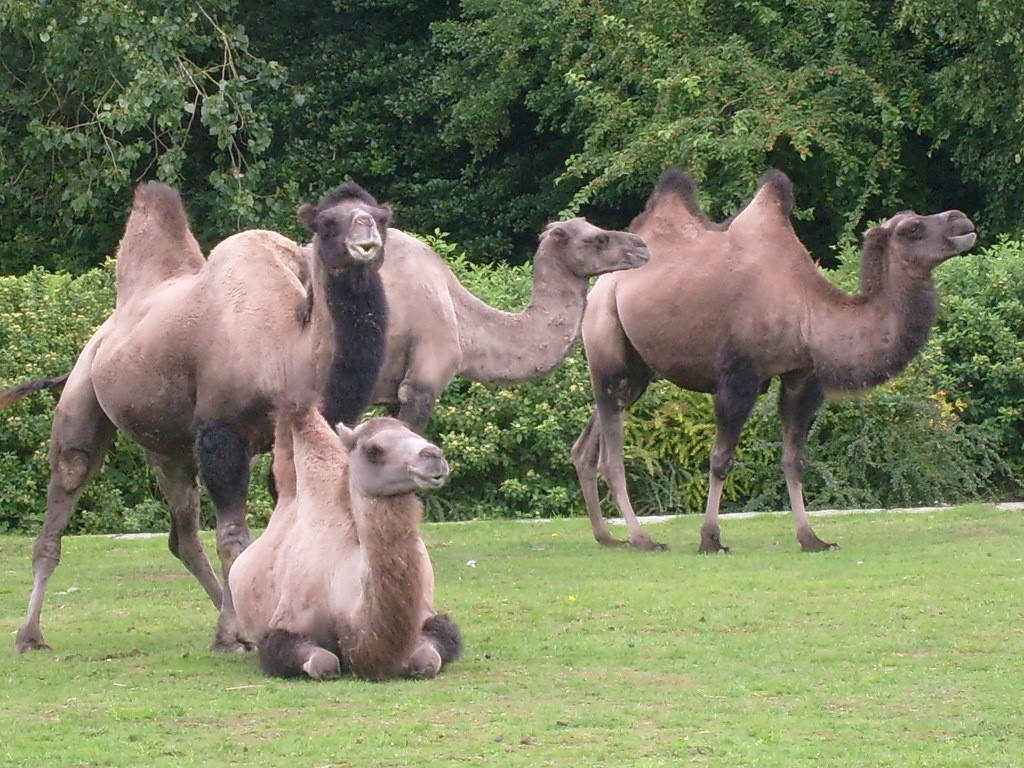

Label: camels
[570,170,977,554]
[15,179,652,683]
